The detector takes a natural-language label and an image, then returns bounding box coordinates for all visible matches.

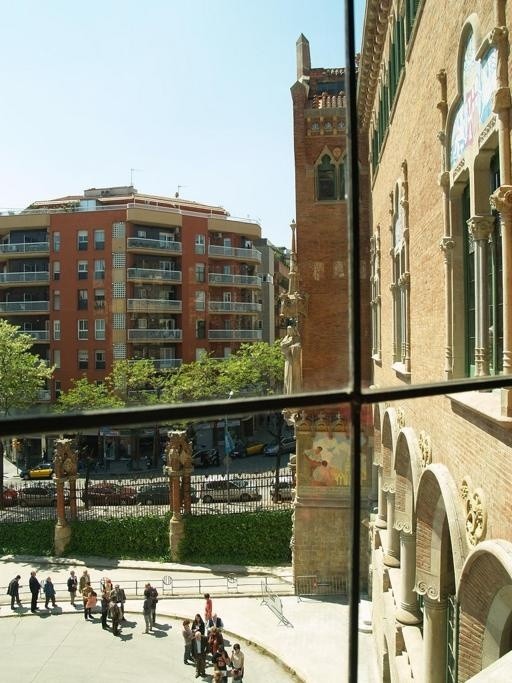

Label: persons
[303,445,322,476]
[7,574,22,609]
[42,449,48,459]
[29,570,42,612]
[183,613,245,682]
[67,570,77,607]
[314,460,331,483]
[280,323,302,394]
[80,569,126,635]
[146,582,158,622]
[204,592,212,624]
[44,577,57,608]
[142,589,152,633]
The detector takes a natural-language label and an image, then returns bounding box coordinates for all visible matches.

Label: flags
[224,431,235,454]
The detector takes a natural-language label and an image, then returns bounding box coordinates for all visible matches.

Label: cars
[191,448,222,468]
[201,479,262,503]
[126,453,152,470]
[16,483,70,506]
[263,435,296,456]
[81,483,137,505]
[20,463,57,479]
[3,484,17,507]
[137,483,197,505]
[230,440,268,460]
[271,476,292,500]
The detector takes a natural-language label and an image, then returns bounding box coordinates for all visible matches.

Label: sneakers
[45,605,58,610]
[195,671,209,678]
[183,656,195,664]
[31,605,40,614]
[84,616,95,620]
